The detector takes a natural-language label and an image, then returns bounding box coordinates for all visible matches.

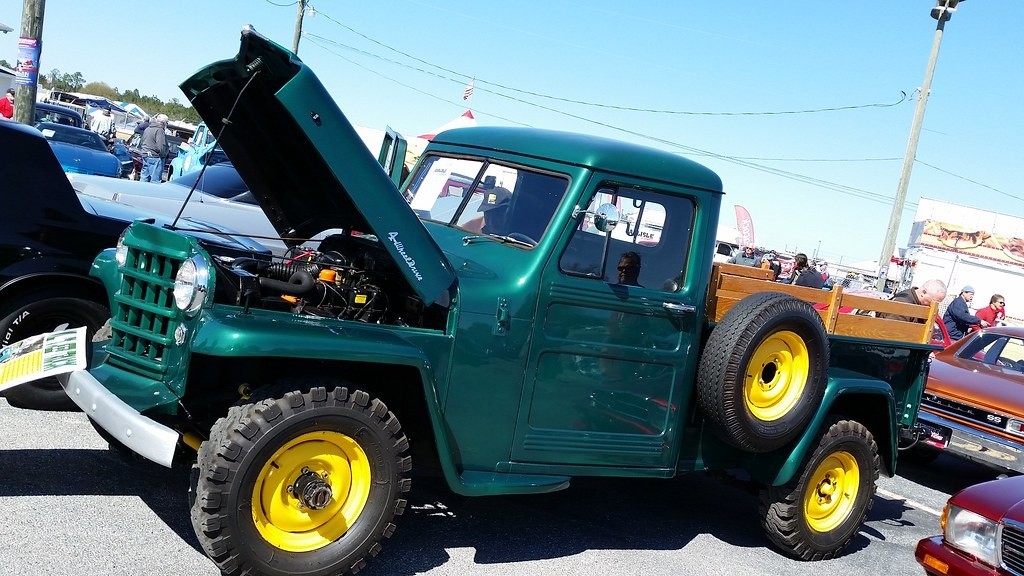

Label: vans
[600,209,738,264]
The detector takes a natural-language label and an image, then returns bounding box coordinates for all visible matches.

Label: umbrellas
[114,99,148,130]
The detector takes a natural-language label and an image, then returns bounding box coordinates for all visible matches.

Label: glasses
[617,265,637,270]
[746,247,752,250]
[997,301,1005,305]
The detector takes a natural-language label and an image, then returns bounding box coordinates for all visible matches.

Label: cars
[812,289,1024,474]
[914,475,1024,576]
[1,103,486,411]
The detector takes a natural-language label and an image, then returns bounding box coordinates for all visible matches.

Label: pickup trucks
[56,24,944,576]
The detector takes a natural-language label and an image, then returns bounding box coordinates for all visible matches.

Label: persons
[460,187,513,237]
[761,253,782,278]
[140,114,171,183]
[785,253,825,288]
[842,271,855,289]
[970,293,1005,350]
[728,245,761,267]
[89,105,117,140]
[939,286,991,341]
[817,260,830,282]
[616,251,646,287]
[127,115,151,175]
[878,279,946,325]
[0,87,15,121]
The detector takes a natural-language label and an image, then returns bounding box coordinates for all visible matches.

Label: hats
[7,88,15,95]
[959,286,974,295]
[476,187,511,212]
[763,254,775,260]
[157,114,169,123]
[105,106,111,112]
[818,261,828,266]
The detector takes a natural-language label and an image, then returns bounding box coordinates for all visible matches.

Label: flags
[463,80,474,100]
